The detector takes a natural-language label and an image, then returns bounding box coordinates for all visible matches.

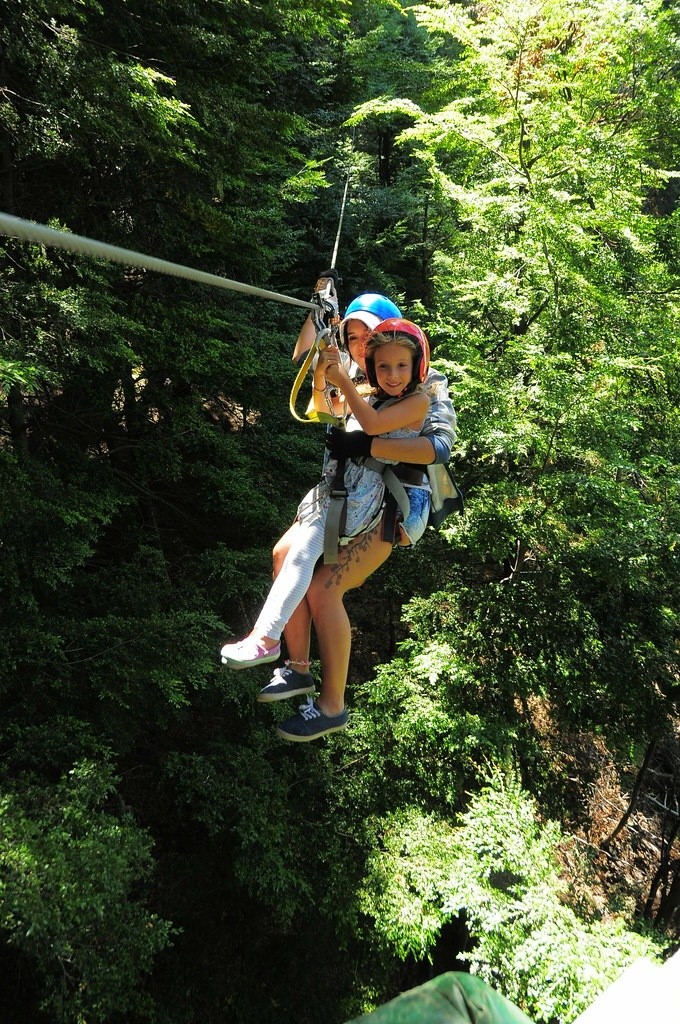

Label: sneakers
[221,658,261,670]
[257,667,316,702]
[221,637,280,663]
[277,695,348,742]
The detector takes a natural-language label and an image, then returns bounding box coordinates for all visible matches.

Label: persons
[220,318,431,670]
[256,276,459,742]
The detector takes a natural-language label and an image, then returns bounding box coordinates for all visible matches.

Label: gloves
[319,269,343,287]
[324,426,379,459]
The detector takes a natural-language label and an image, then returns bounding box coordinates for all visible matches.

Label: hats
[339,311,383,344]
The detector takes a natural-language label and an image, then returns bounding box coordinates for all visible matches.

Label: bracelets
[312,381,327,393]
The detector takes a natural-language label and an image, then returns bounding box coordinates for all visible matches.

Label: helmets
[364,318,430,387]
[344,294,402,321]
